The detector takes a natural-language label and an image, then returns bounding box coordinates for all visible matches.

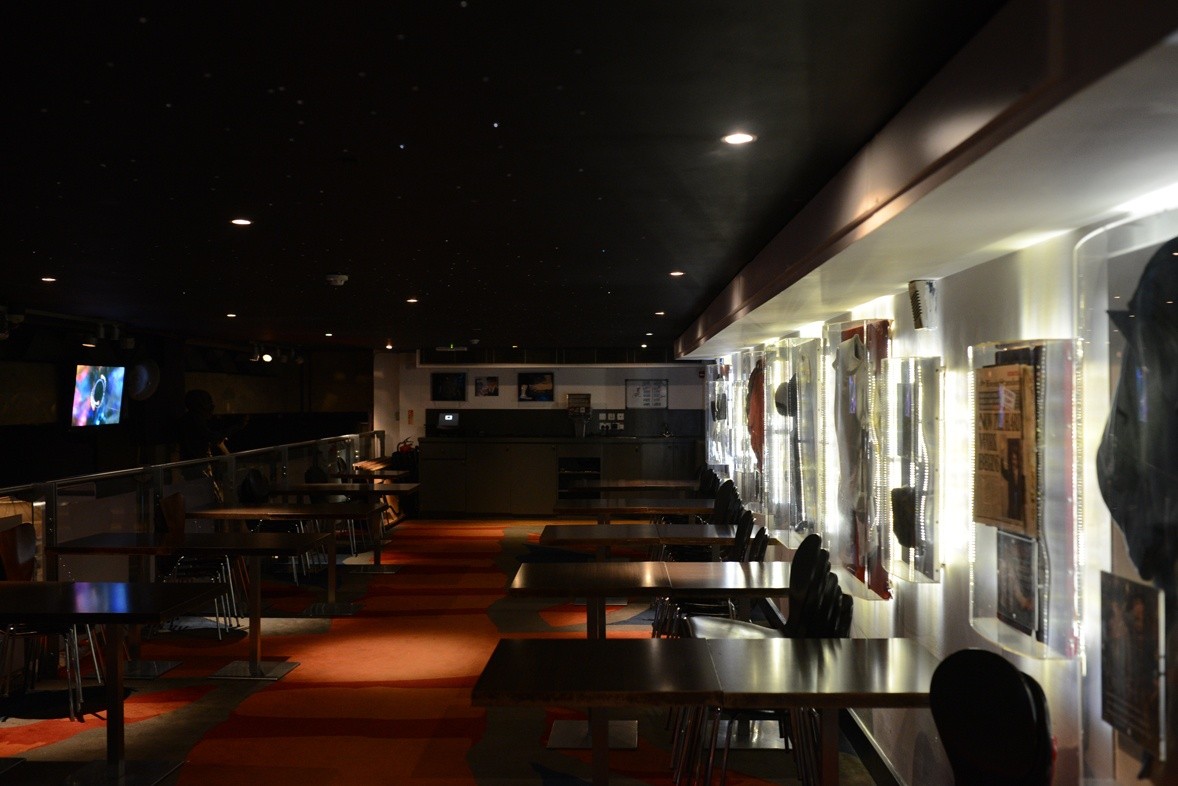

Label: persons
[999,448,1027,522]
[178,390,230,461]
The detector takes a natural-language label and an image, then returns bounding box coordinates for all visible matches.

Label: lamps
[81,332,97,347]
[97,323,105,338]
[119,336,135,350]
[248,344,260,362]
[260,345,273,362]
[110,324,119,340]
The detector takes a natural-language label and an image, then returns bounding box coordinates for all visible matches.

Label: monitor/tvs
[69,362,127,428]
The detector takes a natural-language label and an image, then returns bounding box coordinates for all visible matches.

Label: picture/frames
[517,372,554,402]
[624,379,668,408]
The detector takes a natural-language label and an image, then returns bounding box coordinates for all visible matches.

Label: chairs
[0,456,1056,786]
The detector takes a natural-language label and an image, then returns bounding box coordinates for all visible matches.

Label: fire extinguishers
[400,436,414,480]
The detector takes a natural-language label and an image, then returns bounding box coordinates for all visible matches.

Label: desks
[48,533,331,681]
[540,524,662,638]
[509,561,671,749]
[665,561,794,751]
[550,499,648,605]
[333,470,406,546]
[187,503,388,618]
[639,480,699,498]
[470,638,724,786]
[267,483,422,574]
[706,637,942,786]
[570,479,642,562]
[648,498,718,524]
[0,581,230,786]
[656,524,776,637]
